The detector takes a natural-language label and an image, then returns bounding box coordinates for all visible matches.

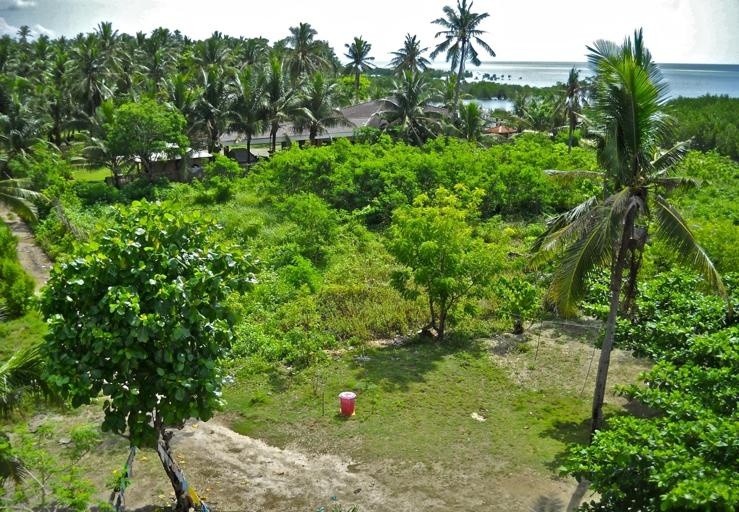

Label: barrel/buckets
[339,391,357,416]
